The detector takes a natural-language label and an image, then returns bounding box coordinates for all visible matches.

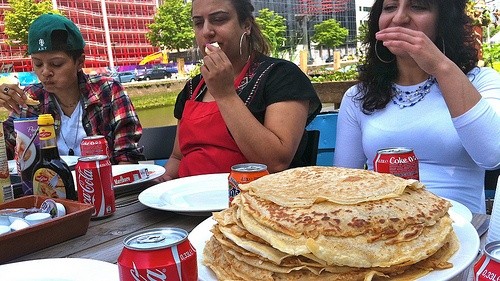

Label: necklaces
[60,97,80,155]
[391,77,435,109]
[59,100,77,107]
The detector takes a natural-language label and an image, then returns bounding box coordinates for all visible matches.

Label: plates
[188,196,480,281]
[138,172,228,216]
[71,164,166,194]
[0,258,119,281]
[8,156,80,175]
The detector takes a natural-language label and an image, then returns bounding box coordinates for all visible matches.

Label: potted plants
[308,70,360,103]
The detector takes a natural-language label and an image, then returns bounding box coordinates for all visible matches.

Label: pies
[24,92,40,105]
[203,164,461,281]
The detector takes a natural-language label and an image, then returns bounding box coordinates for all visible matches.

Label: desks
[1,187,490,281]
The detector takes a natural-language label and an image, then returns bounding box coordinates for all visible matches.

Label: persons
[0,13,144,165]
[333,0,500,216]
[136,0,322,193]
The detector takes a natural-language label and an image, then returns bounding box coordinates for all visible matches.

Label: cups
[10,219,31,232]
[0,208,25,225]
[25,213,51,225]
[0,225,10,234]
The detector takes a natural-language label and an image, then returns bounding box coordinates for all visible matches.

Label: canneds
[75,155,116,219]
[471,240,500,281]
[117,228,198,281]
[79,135,109,157]
[228,164,270,207]
[373,147,419,181]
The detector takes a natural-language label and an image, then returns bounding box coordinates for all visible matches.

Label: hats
[28,13,85,55]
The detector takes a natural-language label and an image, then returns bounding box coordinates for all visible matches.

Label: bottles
[31,114,75,200]
[486,175,500,244]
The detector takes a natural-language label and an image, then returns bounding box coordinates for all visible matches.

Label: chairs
[138,125,177,160]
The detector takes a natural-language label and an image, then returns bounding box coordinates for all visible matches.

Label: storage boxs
[0,195,95,262]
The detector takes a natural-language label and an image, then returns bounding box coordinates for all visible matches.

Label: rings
[3,86,10,94]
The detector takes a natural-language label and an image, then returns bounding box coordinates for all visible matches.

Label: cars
[137,69,172,81]
[109,72,136,82]
